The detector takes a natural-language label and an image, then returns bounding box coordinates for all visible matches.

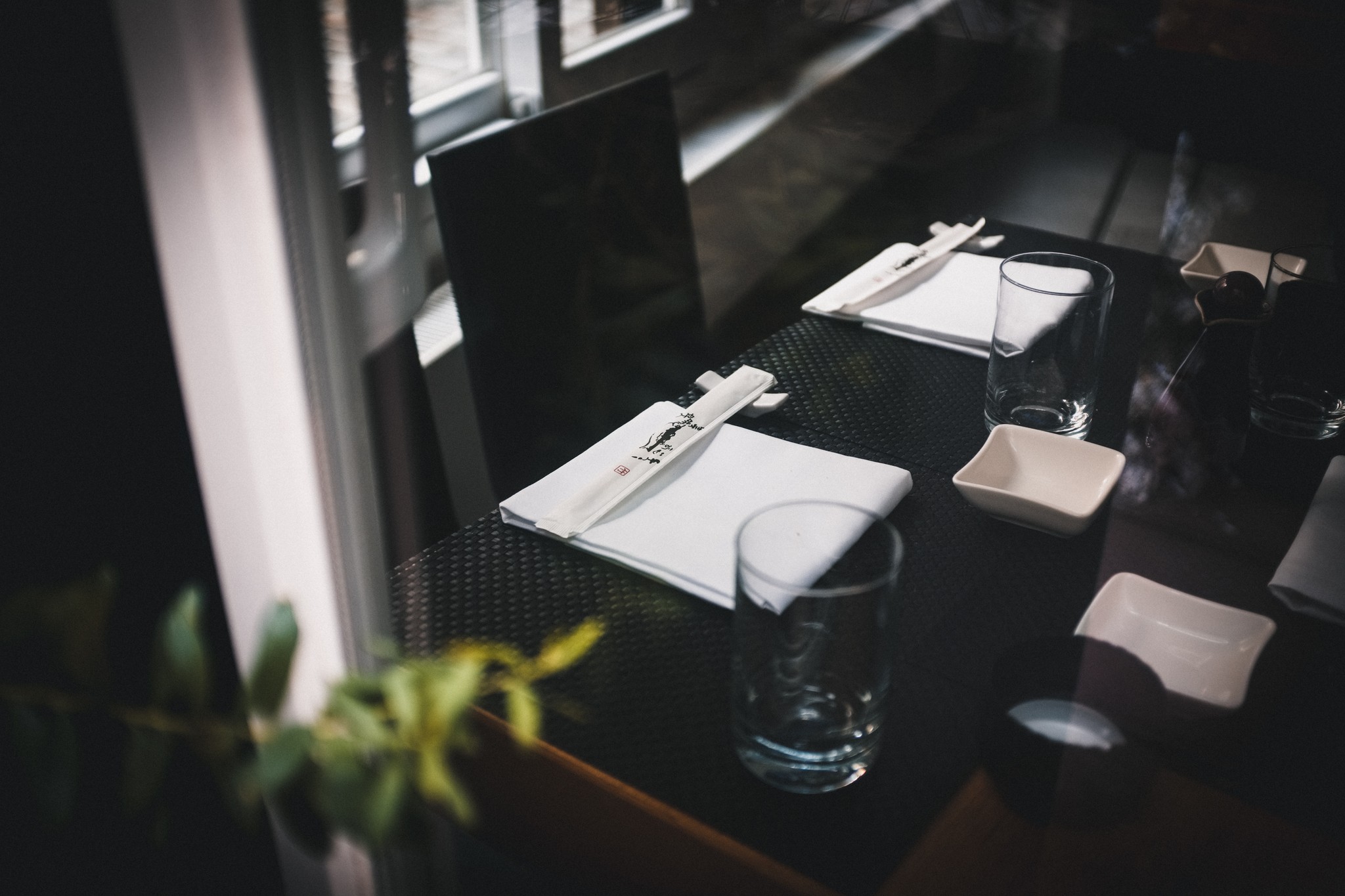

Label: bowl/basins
[1072,570,1276,711]
[1180,241,1306,303]
[952,424,1127,538]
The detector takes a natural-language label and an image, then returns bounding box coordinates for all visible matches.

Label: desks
[382,218,1345,896]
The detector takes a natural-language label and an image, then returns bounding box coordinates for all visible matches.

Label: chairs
[429,69,708,503]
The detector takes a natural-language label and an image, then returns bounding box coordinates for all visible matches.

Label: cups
[983,250,1116,442]
[728,497,904,795]
[1244,242,1345,441]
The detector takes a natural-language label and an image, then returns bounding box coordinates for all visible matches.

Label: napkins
[1267,456,1345,626]
[498,399,912,614]
[800,242,1092,359]
